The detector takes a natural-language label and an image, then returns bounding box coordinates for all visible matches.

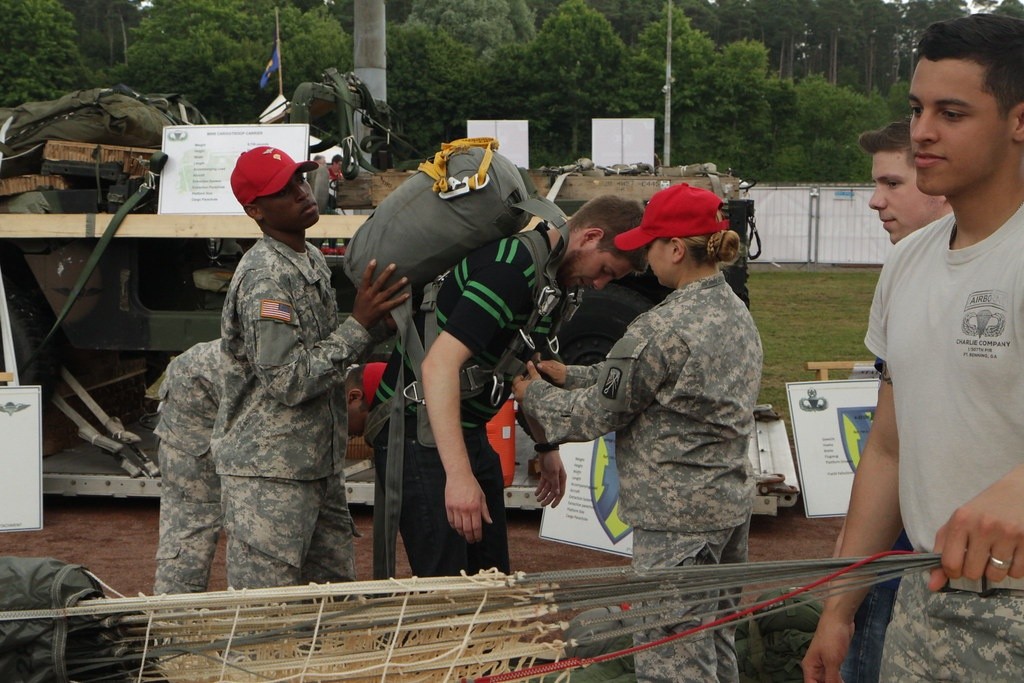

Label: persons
[366,194,649,576]
[802,15,1024,683]
[511,181,764,683]
[209,146,410,658]
[152,336,387,615]
[831,116,955,682]
[314,154,343,212]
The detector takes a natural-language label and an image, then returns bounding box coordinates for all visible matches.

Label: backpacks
[344,138,571,449]
[0,83,210,181]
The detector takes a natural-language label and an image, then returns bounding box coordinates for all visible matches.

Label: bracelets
[534,443,560,453]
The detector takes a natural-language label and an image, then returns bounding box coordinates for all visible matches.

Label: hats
[614,182,729,251]
[363,361,389,407]
[229,146,319,206]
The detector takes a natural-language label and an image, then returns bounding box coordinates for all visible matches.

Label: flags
[260,38,278,88]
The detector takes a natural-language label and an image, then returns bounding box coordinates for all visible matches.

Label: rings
[989,557,1012,570]
